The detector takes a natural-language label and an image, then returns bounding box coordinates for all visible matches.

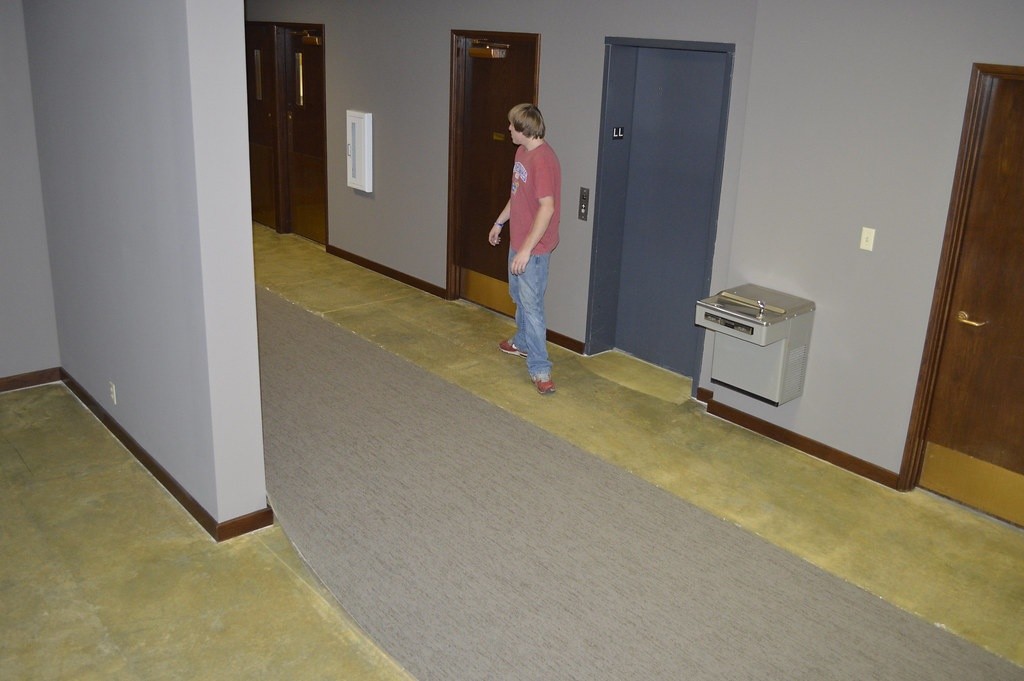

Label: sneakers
[498,338,528,359]
[532,373,556,393]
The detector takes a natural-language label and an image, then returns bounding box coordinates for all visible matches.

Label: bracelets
[494,222,504,228]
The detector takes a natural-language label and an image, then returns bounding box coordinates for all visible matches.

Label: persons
[488,103,561,395]
